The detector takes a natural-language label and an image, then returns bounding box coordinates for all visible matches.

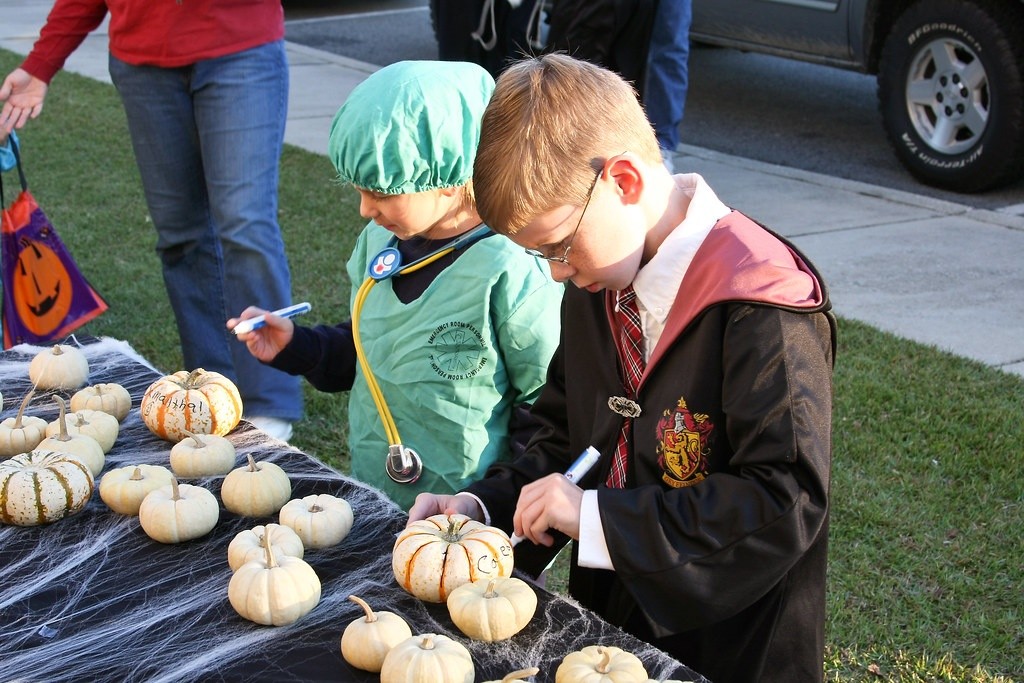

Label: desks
[0,333,711,683]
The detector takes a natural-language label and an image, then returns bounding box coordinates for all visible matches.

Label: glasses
[524,151,627,266]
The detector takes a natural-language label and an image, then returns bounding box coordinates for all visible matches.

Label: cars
[561,0,1024,197]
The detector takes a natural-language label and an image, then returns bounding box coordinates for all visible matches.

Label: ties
[605,283,645,490]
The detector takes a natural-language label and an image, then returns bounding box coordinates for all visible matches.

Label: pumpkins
[0,345,650,683]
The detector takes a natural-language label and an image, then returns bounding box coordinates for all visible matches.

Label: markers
[231,301,312,335]
[510,444,601,547]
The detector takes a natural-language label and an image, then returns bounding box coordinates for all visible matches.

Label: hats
[328,59,496,194]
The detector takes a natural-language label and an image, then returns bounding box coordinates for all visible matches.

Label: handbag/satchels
[0,133,110,351]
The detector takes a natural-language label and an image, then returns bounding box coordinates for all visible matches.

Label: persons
[641,0,692,175]
[473,50,839,683]
[0,0,302,447]
[224,59,566,515]
